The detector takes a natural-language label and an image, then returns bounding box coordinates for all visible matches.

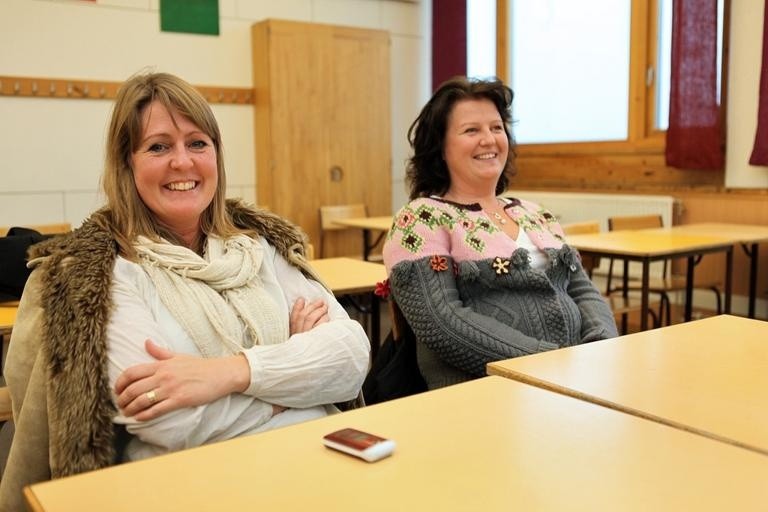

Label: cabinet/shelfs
[249,17,393,259]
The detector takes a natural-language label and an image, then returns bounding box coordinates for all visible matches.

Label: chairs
[0,221,72,239]
[318,204,371,259]
[557,221,661,336]
[609,213,725,327]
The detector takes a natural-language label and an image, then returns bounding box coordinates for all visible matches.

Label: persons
[1,72,374,512]
[382,76,619,391]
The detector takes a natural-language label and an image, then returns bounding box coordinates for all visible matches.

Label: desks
[23,373,768,512]
[333,218,394,260]
[563,222,768,323]
[485,311,768,453]
[2,257,394,421]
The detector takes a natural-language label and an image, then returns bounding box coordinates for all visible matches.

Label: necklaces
[478,196,506,226]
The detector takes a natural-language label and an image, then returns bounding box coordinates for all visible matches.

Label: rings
[146,390,158,403]
